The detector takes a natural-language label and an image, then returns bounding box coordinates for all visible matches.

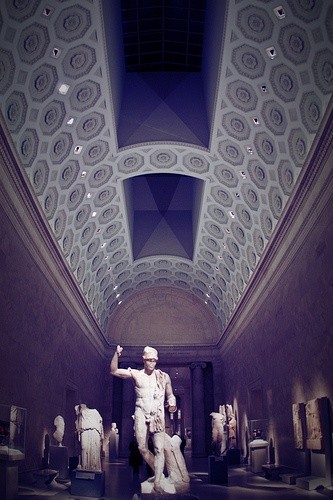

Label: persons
[110,344,177,493]
[47,387,241,459]
